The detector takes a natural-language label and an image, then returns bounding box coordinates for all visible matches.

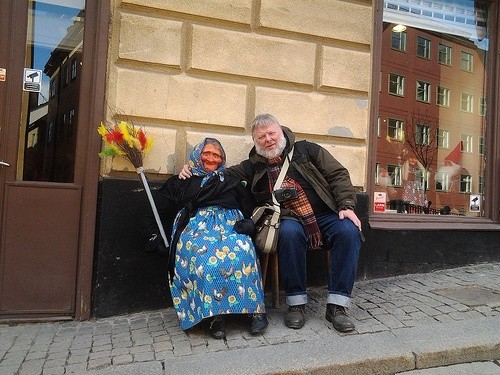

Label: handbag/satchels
[251,203,281,254]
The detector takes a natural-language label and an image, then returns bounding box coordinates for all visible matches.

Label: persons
[146,137,269,339]
[178,113,365,332]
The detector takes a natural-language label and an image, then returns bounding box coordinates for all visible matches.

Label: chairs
[263,247,331,309]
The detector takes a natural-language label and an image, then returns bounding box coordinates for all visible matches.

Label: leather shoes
[249,312,270,333]
[285,302,307,329]
[326,303,355,332]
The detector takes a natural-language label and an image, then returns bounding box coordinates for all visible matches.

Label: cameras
[274,186,299,202]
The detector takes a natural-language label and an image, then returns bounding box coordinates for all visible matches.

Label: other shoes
[208,317,227,340]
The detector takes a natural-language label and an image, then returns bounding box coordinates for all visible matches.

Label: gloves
[153,243,169,260]
[234,219,254,235]
